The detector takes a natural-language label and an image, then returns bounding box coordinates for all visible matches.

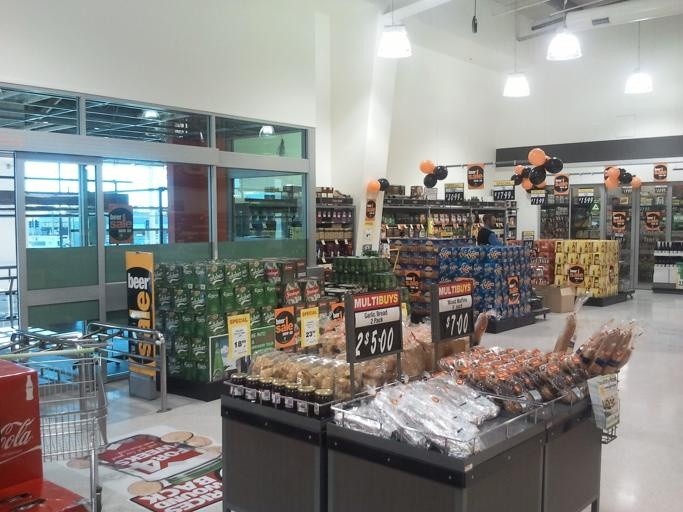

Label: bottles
[317,211,351,227]
[25,375,34,400]
[433,213,468,224]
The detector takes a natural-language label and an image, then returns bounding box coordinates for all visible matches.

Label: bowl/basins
[252,214,274,230]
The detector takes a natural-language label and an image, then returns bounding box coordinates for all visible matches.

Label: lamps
[546,1,583,62]
[624,23,652,94]
[378,1,412,58]
[502,0,530,99]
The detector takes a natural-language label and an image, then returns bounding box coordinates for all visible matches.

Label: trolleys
[0,345,112,511]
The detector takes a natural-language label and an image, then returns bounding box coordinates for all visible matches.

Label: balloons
[378,178,389,192]
[420,160,435,174]
[605,167,641,189]
[367,180,380,193]
[433,166,447,180]
[511,147,563,190]
[424,174,437,188]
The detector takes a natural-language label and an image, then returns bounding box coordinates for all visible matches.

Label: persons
[478,214,502,246]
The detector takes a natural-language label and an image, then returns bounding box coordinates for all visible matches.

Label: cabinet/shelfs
[474,202,519,243]
[381,186,473,243]
[315,187,356,267]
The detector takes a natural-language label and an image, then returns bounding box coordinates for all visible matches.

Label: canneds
[229,373,246,399]
[315,388,333,419]
[258,377,273,407]
[245,375,259,403]
[284,383,298,412]
[272,379,286,409]
[297,386,315,417]
